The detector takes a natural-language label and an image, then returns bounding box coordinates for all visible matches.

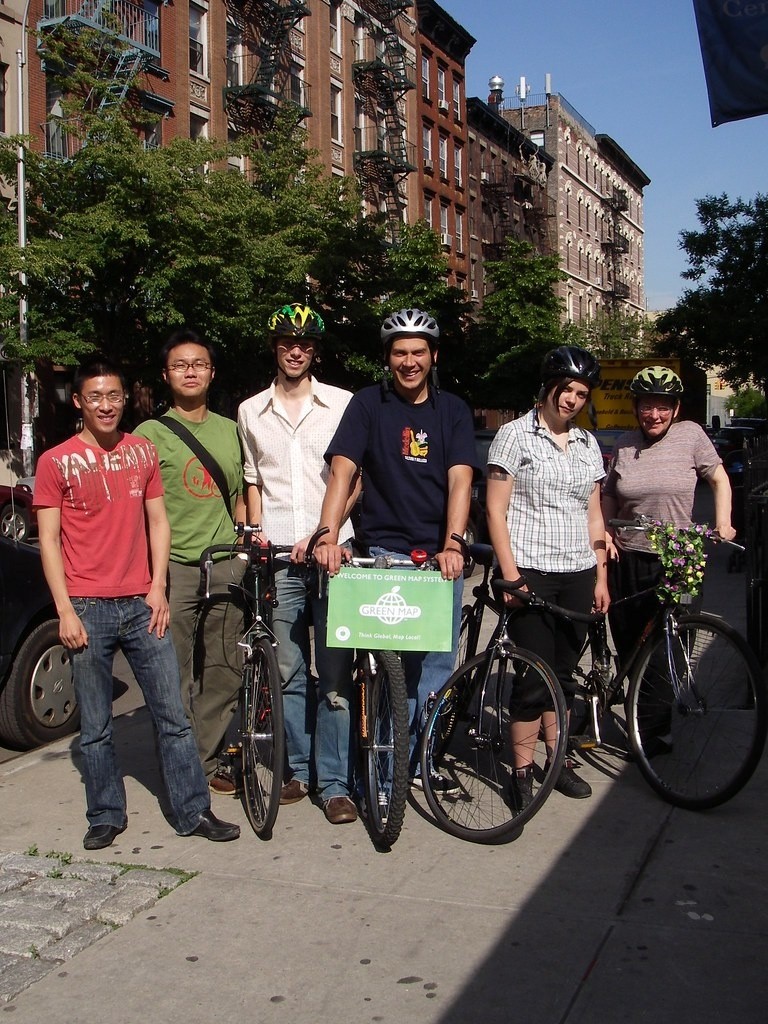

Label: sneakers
[410,770,461,795]
[361,789,388,823]
[278,780,315,804]
[512,765,533,812]
[541,759,592,799]
[319,797,358,823]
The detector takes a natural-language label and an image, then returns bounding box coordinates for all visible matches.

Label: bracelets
[237,553,251,568]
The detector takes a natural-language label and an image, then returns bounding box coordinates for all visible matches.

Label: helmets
[267,303,325,344]
[380,308,440,350]
[630,365,683,399]
[541,345,600,390]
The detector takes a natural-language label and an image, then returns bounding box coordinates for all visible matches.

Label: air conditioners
[438,100,449,111]
[595,277,601,284]
[440,232,453,246]
[471,290,478,298]
[480,171,490,184]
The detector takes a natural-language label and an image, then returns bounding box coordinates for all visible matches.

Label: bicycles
[303,521,476,849]
[571,516,768,812]
[199,520,360,835]
[418,544,607,842]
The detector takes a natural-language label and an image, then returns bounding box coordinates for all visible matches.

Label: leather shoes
[83,824,127,849]
[192,809,241,841]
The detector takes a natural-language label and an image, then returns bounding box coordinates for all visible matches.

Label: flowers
[640,516,713,603]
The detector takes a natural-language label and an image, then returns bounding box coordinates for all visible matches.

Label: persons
[315,307,483,825]
[601,366,738,761]
[484,346,610,819]
[237,303,363,824]
[31,358,241,851]
[129,331,251,795]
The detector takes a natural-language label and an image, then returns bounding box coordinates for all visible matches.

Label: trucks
[575,357,708,431]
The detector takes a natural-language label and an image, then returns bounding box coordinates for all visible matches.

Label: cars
[15,476,36,496]
[0,485,40,543]
[471,428,499,507]
[0,532,82,749]
[701,417,768,493]
[586,428,628,472]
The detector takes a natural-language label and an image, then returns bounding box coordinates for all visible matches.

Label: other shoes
[626,738,674,760]
[209,776,236,794]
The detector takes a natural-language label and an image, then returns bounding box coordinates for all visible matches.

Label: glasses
[167,361,212,371]
[80,392,124,404]
[638,404,674,415]
